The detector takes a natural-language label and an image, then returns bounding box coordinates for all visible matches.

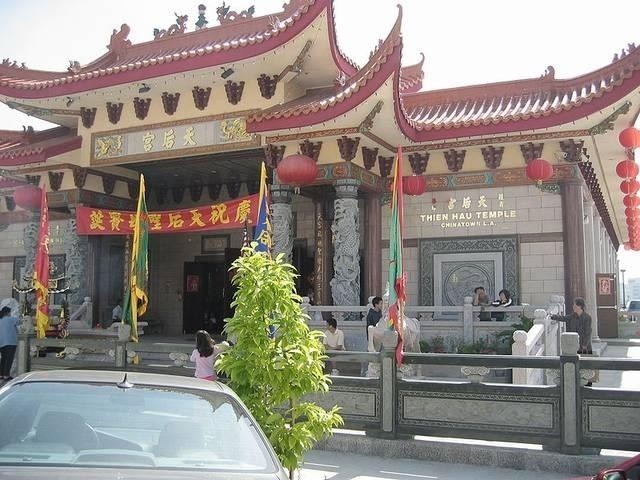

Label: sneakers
[0,375,13,381]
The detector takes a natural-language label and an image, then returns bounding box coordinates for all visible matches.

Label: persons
[472,286,491,321]
[190,330,230,381]
[0,306,20,381]
[492,289,513,321]
[549,297,593,387]
[365,296,383,338]
[112,299,123,331]
[322,317,347,376]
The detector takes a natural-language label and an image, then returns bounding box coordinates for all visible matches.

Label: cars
[563,451,640,480]
[622,299,640,321]
[0,367,291,480]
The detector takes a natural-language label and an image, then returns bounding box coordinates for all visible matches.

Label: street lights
[620,269,628,308]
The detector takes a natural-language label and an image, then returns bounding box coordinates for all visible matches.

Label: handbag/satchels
[580,348,599,383]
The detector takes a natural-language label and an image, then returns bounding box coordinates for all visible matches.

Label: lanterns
[276,153,319,194]
[403,173,426,197]
[526,156,553,186]
[616,127,640,253]
[13,182,49,211]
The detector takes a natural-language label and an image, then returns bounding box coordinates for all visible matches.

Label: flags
[122,174,148,342]
[37,184,51,338]
[253,160,276,370]
[387,156,407,367]
